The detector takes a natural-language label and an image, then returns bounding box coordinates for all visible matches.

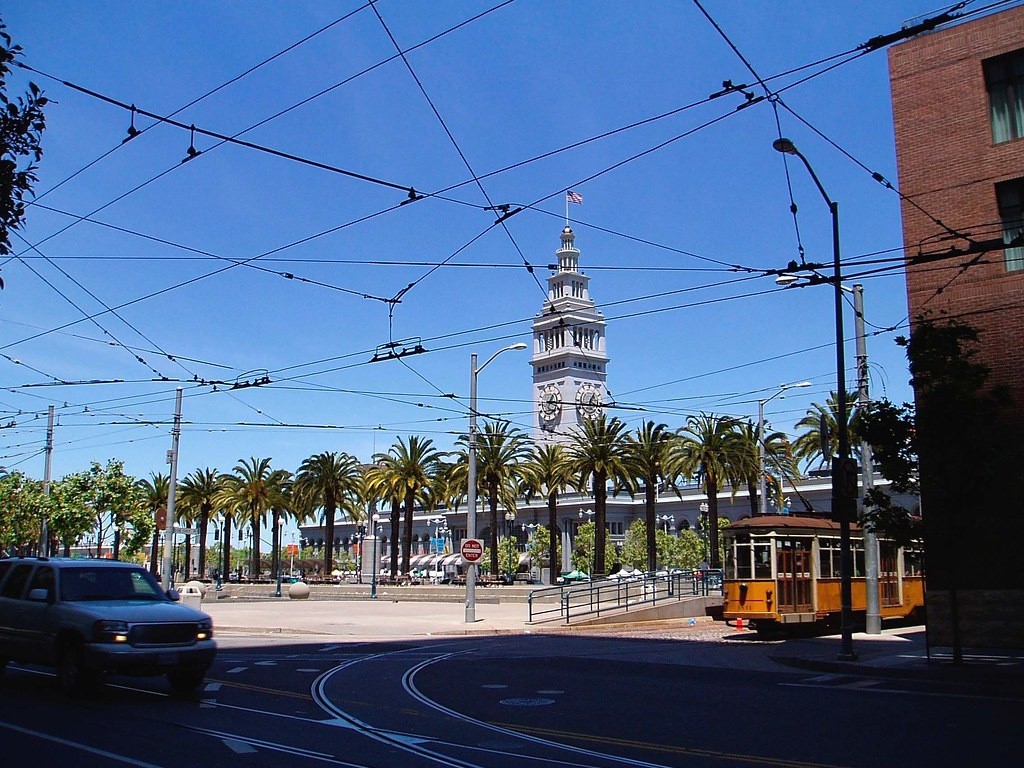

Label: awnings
[381,552,529,565]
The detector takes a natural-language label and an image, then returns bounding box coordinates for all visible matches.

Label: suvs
[0,554,218,701]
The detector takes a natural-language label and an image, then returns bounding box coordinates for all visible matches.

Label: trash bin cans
[175,585,202,610]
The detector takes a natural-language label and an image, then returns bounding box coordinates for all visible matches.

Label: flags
[568,191,583,205]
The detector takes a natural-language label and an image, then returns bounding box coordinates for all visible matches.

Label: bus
[717,509,926,636]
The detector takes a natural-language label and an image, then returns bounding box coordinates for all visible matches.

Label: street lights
[773,136,858,658]
[274,517,285,597]
[504,511,516,585]
[521,522,540,583]
[773,274,882,634]
[655,514,675,582]
[579,507,596,583]
[356,518,369,584]
[700,502,710,562]
[760,381,812,564]
[463,342,528,623]
[426,516,448,586]
[370,513,381,599]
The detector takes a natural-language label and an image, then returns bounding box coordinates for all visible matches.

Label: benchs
[454,575,505,587]
[375,575,409,585]
[195,578,213,584]
[241,577,341,585]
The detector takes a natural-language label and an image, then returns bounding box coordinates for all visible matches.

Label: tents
[609,568,648,578]
[562,570,589,584]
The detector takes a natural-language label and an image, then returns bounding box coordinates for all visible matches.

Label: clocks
[576,384,603,420]
[538,386,562,421]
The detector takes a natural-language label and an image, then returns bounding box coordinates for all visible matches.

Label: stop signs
[460,538,484,563]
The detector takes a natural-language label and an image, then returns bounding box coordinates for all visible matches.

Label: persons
[699,559,710,582]
[402,567,427,586]
[379,567,402,576]
